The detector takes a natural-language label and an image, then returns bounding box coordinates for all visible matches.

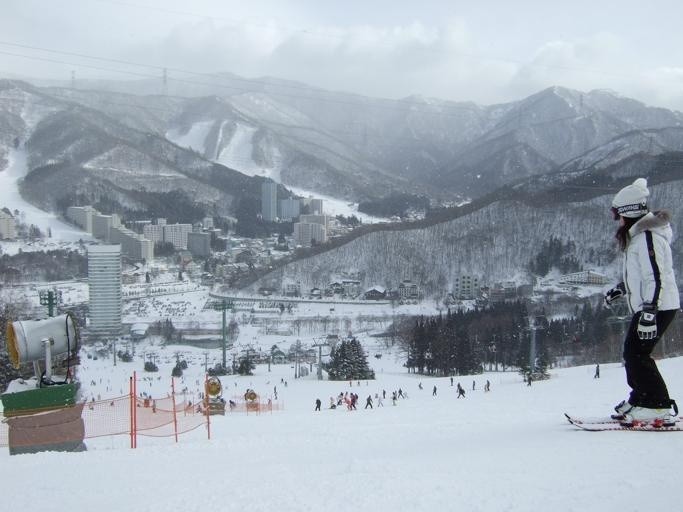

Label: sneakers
[611,398,677,427]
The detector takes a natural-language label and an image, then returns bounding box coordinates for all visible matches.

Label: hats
[611,178,651,219]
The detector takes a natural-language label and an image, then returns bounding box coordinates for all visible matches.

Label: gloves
[637,305,658,339]
[603,282,626,311]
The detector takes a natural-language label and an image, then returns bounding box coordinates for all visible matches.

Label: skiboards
[563,412,682,432]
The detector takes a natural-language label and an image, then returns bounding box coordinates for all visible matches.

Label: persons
[603,178,682,427]
[594,364,600,378]
[527,375,533,386]
[314,374,493,411]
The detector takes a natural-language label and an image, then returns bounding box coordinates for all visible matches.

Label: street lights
[214,299,233,371]
[37,288,62,318]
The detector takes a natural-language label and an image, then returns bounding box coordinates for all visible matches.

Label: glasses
[609,204,647,219]
